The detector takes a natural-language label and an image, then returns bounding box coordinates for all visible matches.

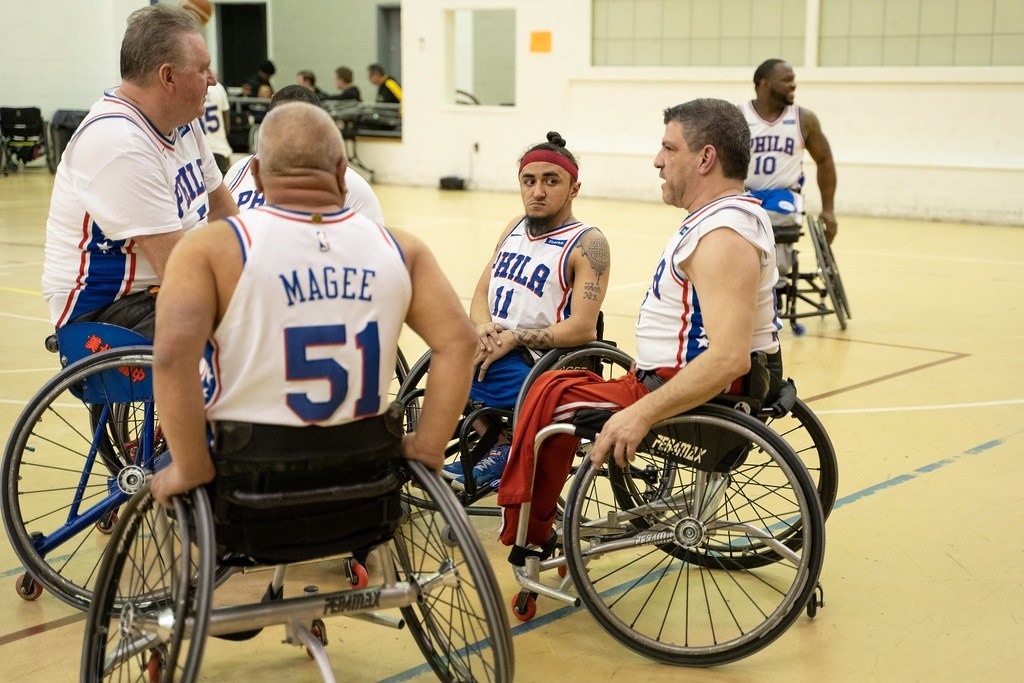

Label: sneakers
[443,457,465,481]
[450,445,510,491]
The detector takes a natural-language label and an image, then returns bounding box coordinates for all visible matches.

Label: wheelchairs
[512,333,838,668]
[774,214,852,330]
[0,107,57,177]
[78,400,514,683]
[0,310,418,621]
[394,309,677,544]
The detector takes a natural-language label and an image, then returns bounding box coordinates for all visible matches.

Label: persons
[224,81,255,152]
[494,97,780,546]
[196,79,238,179]
[435,130,611,491]
[295,69,332,103]
[328,66,363,103]
[711,56,837,320]
[245,84,277,128]
[146,101,481,510]
[369,64,403,102]
[236,60,277,129]
[213,83,390,224]
[43,0,246,407]
